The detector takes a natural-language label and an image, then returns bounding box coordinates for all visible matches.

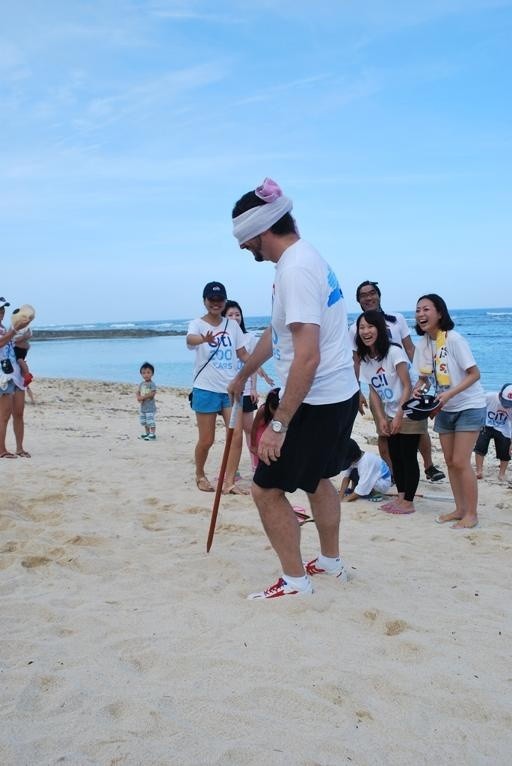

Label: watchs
[271,419,288,434]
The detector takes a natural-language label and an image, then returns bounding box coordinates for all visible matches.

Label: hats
[357,282,381,312]
[203,282,226,301]
[499,383,512,407]
[0,297,10,308]
[403,396,441,420]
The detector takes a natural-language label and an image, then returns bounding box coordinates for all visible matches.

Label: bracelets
[12,325,18,333]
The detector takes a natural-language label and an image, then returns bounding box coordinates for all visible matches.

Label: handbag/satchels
[188,390,192,408]
[1,359,14,374]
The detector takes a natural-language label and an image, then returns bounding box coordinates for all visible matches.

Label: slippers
[195,476,213,492]
[17,451,31,458]
[221,486,250,495]
[0,453,17,458]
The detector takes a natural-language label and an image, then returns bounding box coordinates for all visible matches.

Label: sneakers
[145,432,155,440]
[336,488,352,496]
[138,434,150,439]
[246,577,312,601]
[425,465,445,481]
[22,373,33,378]
[24,375,32,387]
[303,556,347,586]
[367,493,383,501]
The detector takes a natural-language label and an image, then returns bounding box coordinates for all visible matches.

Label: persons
[0,297,30,460]
[184,282,280,496]
[473,382,512,484]
[340,281,487,528]
[225,176,361,601]
[11,304,35,387]
[136,362,158,441]
